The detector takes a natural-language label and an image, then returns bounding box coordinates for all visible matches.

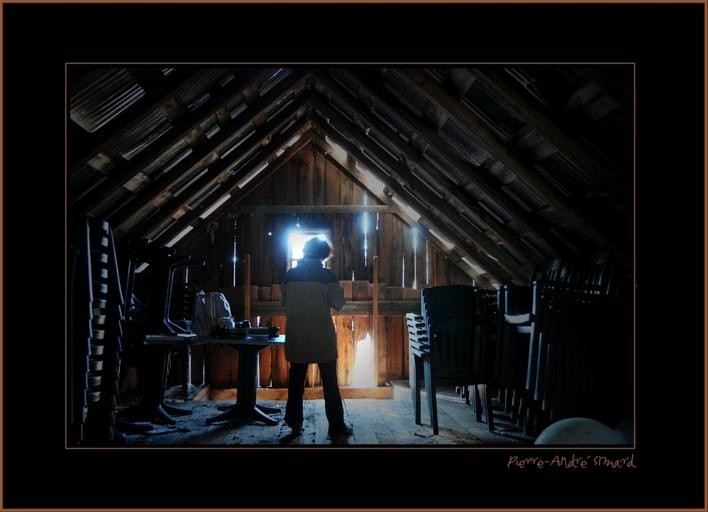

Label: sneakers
[327,423,353,438]
[283,418,303,433]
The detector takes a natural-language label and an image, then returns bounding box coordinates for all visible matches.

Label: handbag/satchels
[194,290,234,336]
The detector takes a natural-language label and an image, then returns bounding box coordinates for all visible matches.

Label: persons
[280,236,354,436]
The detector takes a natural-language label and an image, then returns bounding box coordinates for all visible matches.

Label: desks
[130,332,287,425]
